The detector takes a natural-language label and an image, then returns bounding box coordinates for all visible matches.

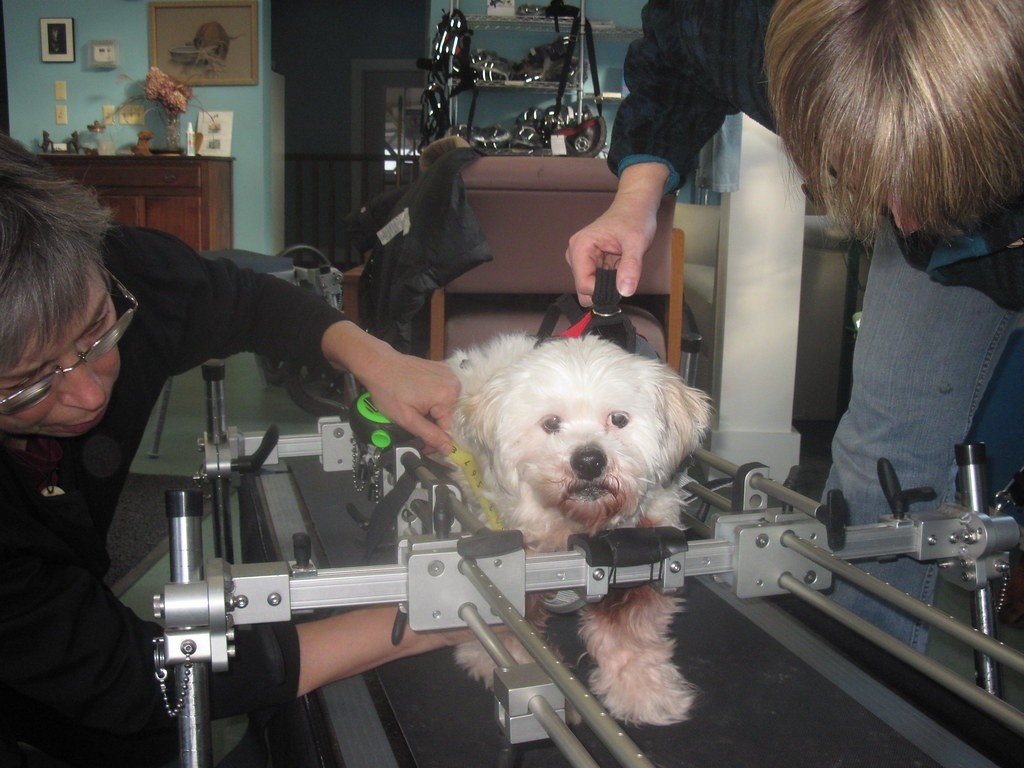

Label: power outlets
[103,105,115,125]
[119,104,145,124]
[55,80,66,100]
[55,105,67,125]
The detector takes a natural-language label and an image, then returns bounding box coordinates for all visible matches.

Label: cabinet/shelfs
[30,154,245,255]
[448,0,644,158]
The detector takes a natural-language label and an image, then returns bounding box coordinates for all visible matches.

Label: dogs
[441,329,719,726]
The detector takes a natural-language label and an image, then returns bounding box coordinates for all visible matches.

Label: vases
[161,113,180,152]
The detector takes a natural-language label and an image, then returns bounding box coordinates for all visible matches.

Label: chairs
[425,156,686,374]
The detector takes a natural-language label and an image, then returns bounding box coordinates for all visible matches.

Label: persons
[564,0,1024,527]
[0,133,509,768]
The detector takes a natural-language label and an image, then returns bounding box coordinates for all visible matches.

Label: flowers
[111,66,221,135]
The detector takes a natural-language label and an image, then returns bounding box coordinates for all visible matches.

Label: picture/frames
[39,16,76,64]
[148,0,259,87]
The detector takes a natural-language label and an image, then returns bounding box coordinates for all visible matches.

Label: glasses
[0,261,139,416]
